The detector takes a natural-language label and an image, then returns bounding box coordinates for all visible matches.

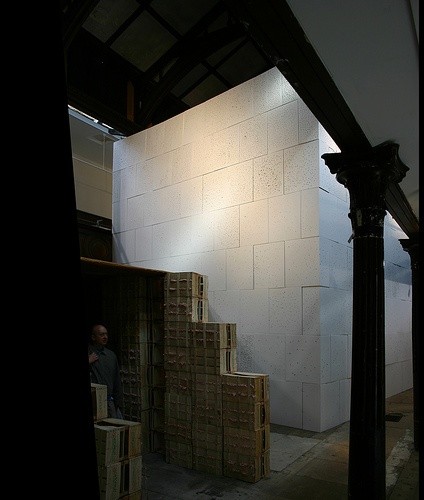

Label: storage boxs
[80,269,271,499]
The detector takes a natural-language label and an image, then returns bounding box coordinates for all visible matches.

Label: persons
[86,324,123,420]
